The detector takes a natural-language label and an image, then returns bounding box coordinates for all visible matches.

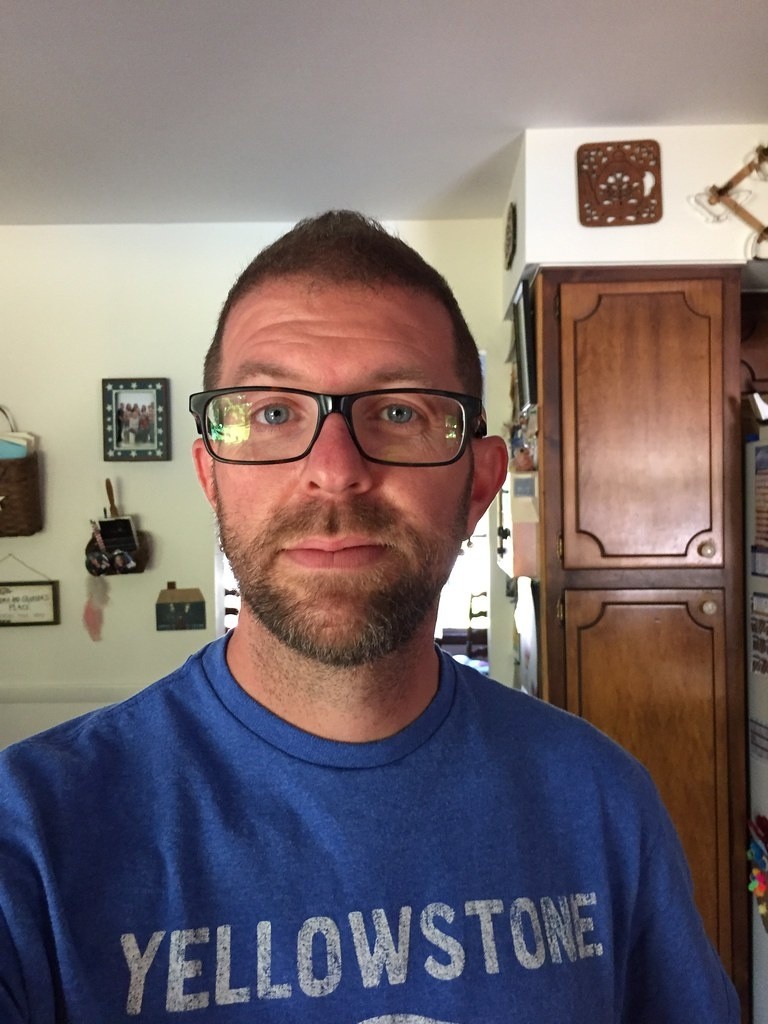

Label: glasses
[188,384,488,467]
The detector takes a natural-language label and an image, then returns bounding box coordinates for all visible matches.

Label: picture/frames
[0,580,61,627]
[511,278,536,416]
[101,377,171,462]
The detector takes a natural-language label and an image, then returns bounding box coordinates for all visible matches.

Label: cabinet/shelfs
[499,263,754,1024]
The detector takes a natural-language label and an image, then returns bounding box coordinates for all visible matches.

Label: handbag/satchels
[0,407,42,537]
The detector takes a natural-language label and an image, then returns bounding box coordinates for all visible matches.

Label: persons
[0,209,741,1024]
[116,402,155,448]
